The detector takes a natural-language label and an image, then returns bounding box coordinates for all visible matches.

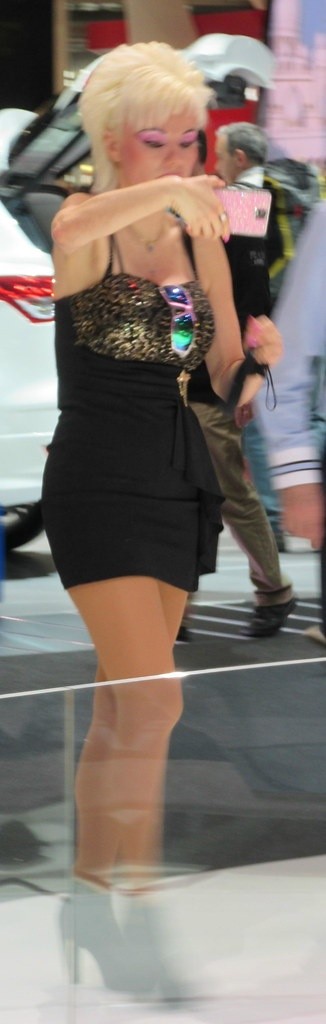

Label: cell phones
[213,188,272,237]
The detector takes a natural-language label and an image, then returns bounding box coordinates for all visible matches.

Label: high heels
[59,882,181,1003]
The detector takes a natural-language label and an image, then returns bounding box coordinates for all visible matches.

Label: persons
[40,39,283,1010]
[177,120,326,642]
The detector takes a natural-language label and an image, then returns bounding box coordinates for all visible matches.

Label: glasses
[160,283,196,360]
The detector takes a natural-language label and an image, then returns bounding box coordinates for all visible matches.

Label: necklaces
[124,227,165,251]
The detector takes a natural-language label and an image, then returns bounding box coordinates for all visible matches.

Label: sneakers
[247,597,297,638]
[175,626,190,644]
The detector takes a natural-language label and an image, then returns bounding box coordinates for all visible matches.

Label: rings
[219,212,227,221]
